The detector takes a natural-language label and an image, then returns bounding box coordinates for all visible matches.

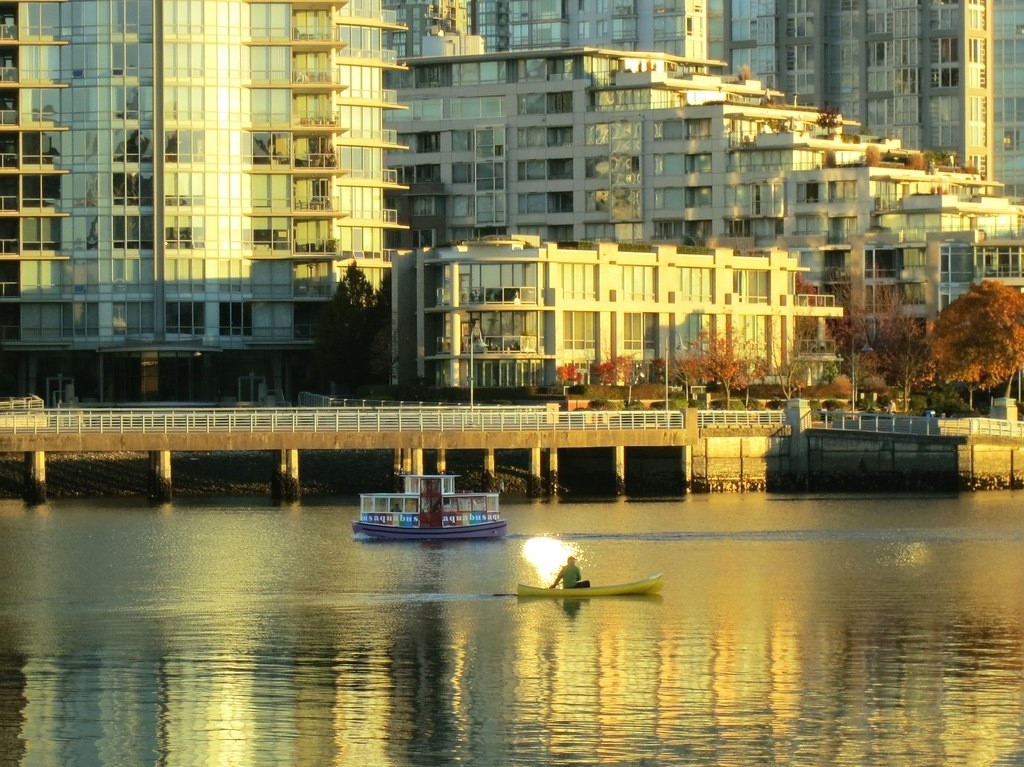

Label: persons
[391,503,402,512]
[885,399,896,414]
[868,406,875,413]
[550,556,590,590]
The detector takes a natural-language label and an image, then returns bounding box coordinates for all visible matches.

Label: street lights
[471,326,487,424]
[851,331,874,420]
[665,329,687,412]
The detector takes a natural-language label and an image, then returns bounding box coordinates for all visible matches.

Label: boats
[349,469,509,539]
[516,574,662,598]
